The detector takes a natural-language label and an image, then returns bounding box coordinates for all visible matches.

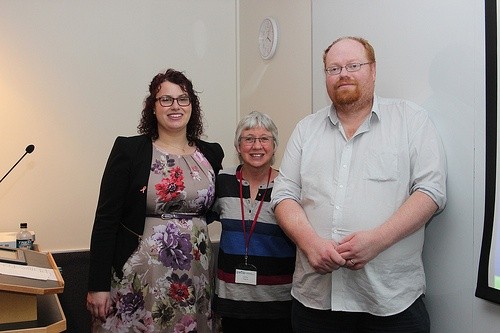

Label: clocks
[258,17,278,60]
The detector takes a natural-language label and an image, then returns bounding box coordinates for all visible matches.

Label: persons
[208,111,296,333]
[87,69,225,333]
[270,38,448,333]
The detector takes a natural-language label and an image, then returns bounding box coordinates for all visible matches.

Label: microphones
[0,144,35,182]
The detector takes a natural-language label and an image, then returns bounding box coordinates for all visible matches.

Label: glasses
[326,62,373,75]
[241,136,274,144]
[156,93,191,107]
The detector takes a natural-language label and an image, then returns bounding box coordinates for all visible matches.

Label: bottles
[16,222,33,251]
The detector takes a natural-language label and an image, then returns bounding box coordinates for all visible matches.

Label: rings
[351,260,355,264]
